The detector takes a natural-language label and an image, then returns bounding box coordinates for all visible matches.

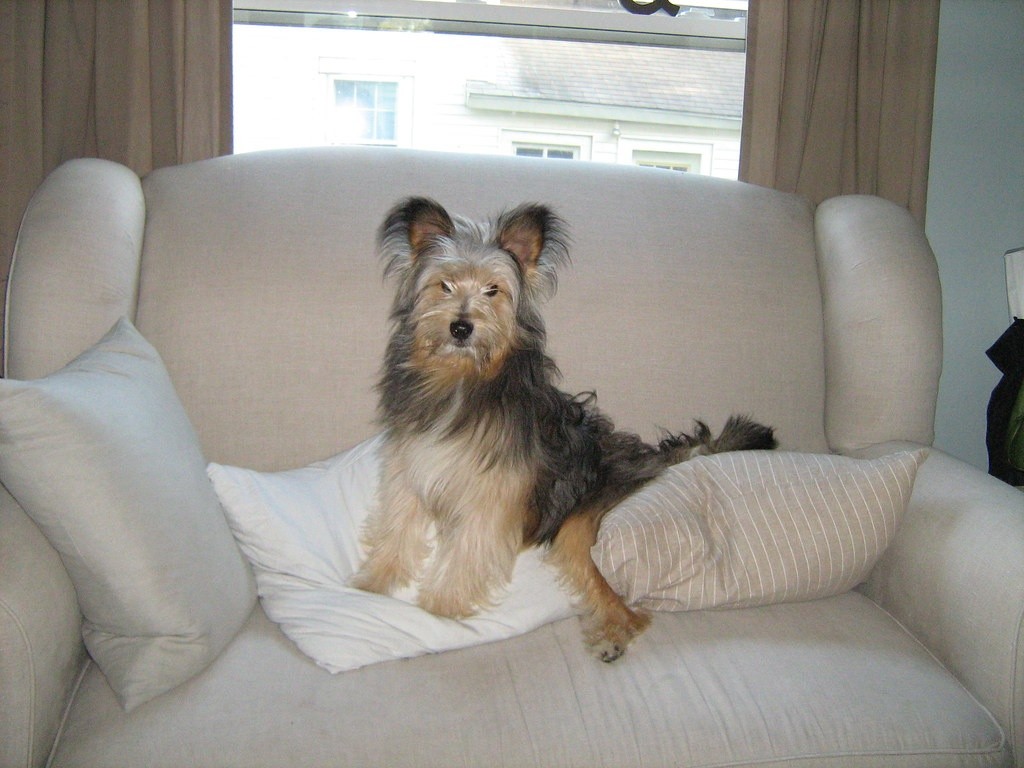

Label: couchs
[0,145,1023,768]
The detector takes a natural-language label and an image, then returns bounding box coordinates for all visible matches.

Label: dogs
[346,196,778,664]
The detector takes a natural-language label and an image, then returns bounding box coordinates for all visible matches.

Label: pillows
[590,449,928,615]
[0,317,260,708]
[208,429,594,677]
